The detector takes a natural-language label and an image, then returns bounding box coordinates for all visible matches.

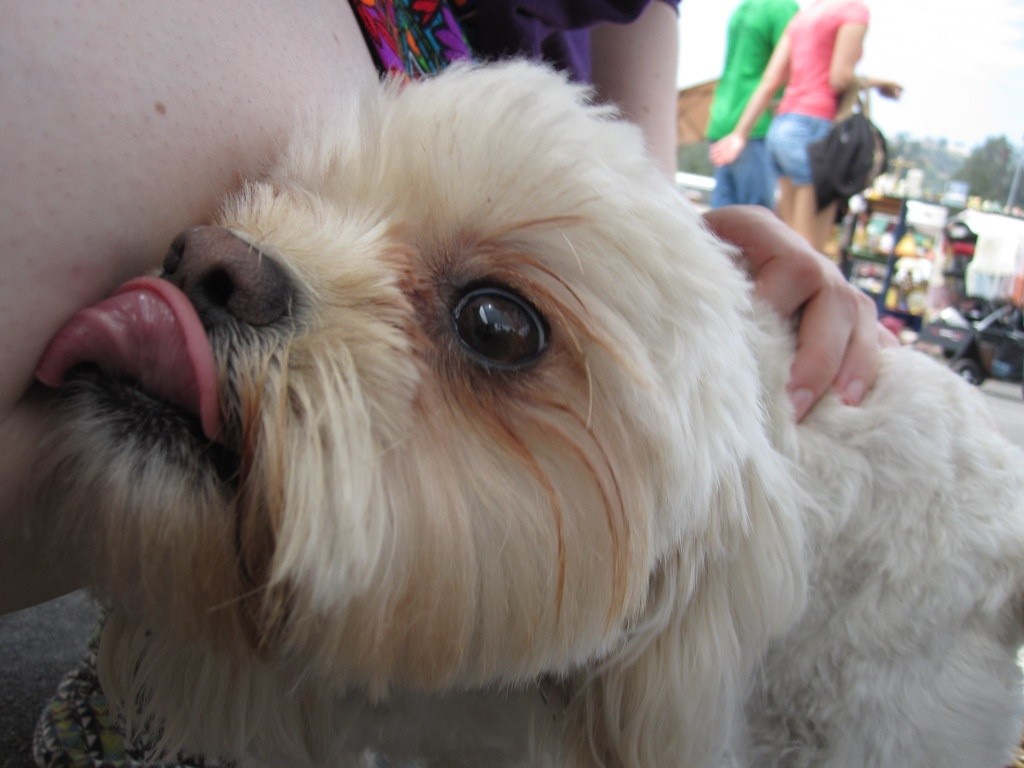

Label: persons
[710,3,904,258]
[704,2,804,209]
[1,0,910,766]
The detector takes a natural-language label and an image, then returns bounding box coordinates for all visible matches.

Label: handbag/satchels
[806,78,886,227]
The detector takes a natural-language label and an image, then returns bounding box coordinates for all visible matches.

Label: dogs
[35,49,1022,768]
